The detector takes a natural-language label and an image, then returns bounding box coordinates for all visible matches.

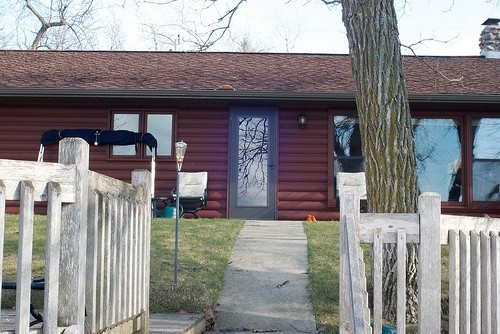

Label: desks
[151,197,168,219]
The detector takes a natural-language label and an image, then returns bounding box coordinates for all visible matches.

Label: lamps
[298,112,307,129]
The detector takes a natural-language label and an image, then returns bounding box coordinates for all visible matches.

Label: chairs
[171,170,209,220]
